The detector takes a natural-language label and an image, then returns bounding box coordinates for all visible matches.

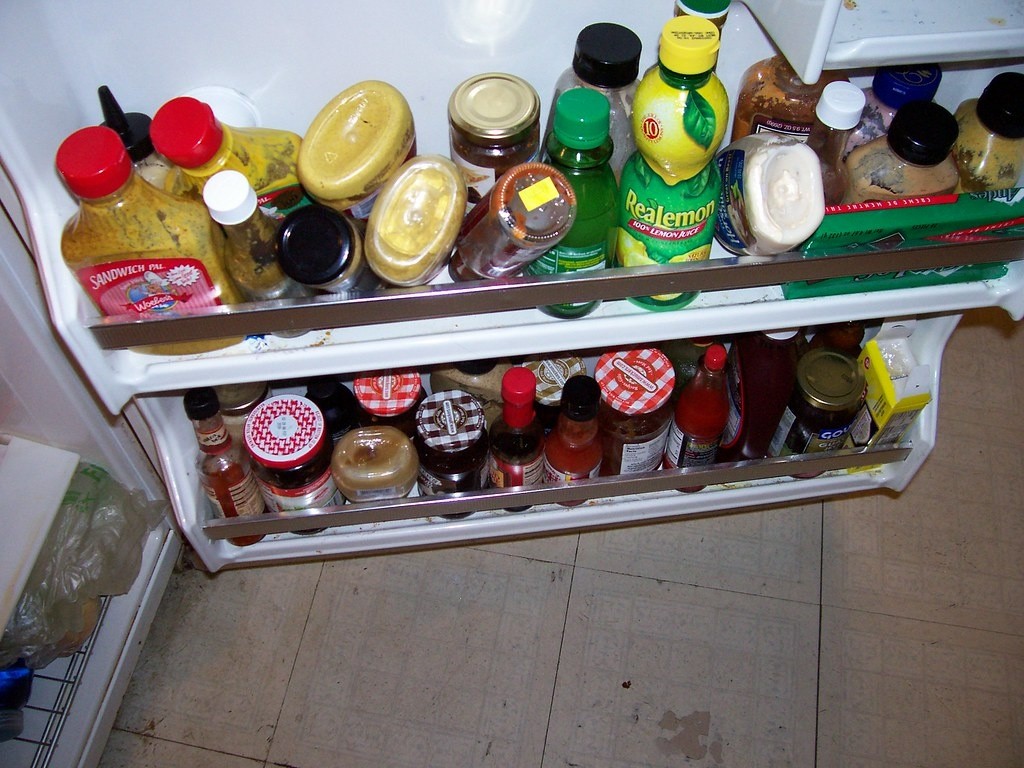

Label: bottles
[331,426,419,503]
[525,0,1024,319]
[183,387,270,546]
[544,375,602,507]
[714,326,810,462]
[430,358,514,431]
[55,0,467,354]
[304,376,362,446]
[663,334,729,492]
[450,164,576,282]
[488,367,545,512]
[808,321,864,356]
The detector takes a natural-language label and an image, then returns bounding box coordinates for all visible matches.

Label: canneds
[354,369,487,520]
[522,357,587,437]
[217,380,346,535]
[767,346,866,479]
[274,203,388,293]
[593,347,677,476]
[448,72,576,282]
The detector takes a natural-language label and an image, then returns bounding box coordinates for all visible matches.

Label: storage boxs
[780,191,1023,299]
